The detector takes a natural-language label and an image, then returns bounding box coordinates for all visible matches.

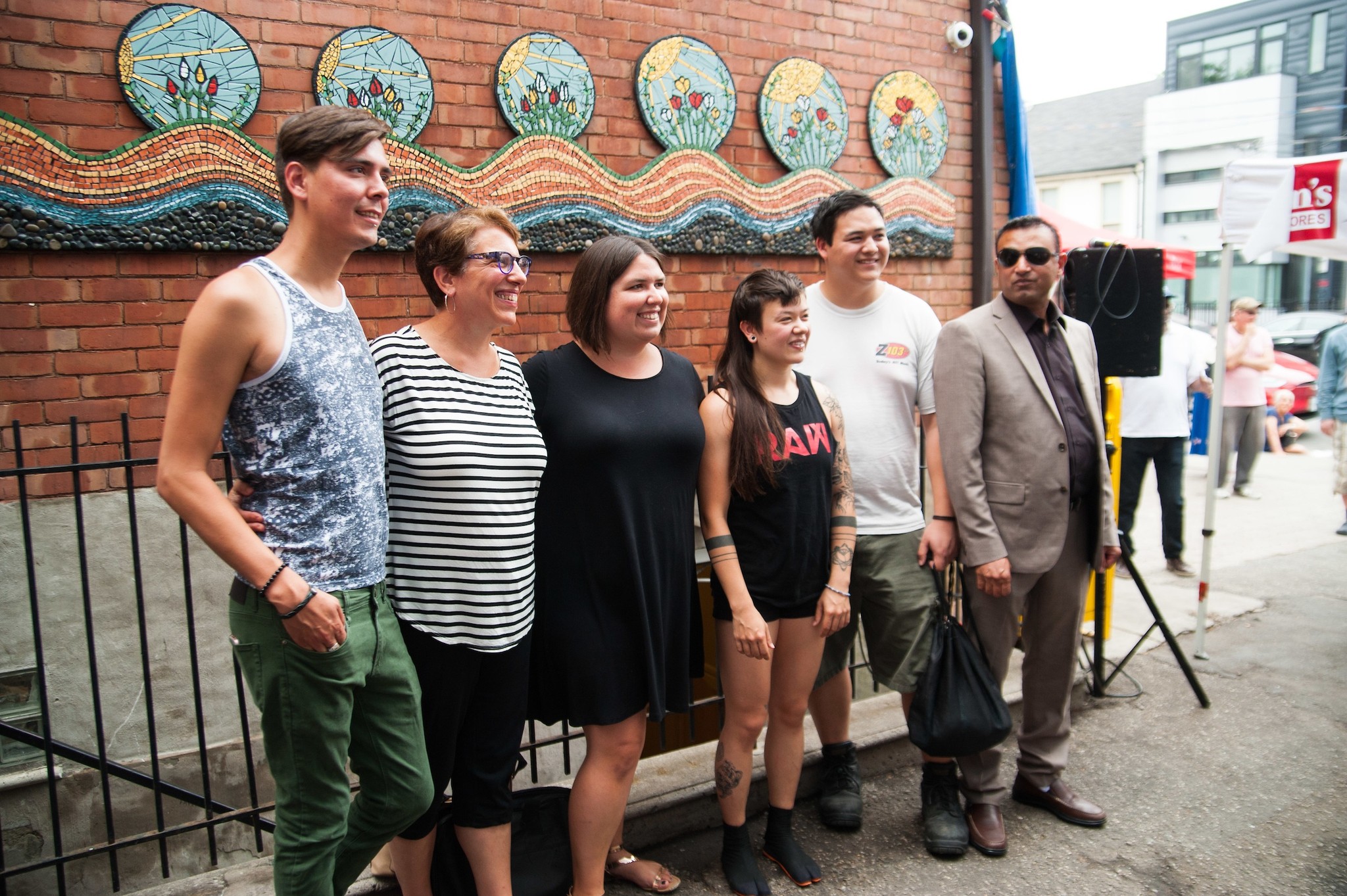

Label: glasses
[1246,310,1258,315]
[463,249,532,276]
[991,247,1059,268]
[1164,302,1169,309]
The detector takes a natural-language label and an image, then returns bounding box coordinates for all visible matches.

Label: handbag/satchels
[907,551,1012,758]
[431,750,572,895]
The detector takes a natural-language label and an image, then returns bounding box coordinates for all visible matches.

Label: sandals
[602,846,681,892]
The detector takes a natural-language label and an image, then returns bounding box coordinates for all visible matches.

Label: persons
[1317,324,1347,536]
[794,190,972,860]
[521,234,707,896]
[225,207,548,896]
[1263,392,1311,455]
[933,217,1122,857]
[154,106,435,896]
[1117,287,1211,580]
[1215,298,1275,500]
[697,269,856,896]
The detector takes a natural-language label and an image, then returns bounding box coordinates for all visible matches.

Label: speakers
[1065,248,1163,377]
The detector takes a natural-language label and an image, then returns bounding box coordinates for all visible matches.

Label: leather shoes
[1011,770,1108,825]
[965,797,1007,856]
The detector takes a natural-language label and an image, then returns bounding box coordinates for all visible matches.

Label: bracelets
[278,585,318,621]
[825,584,851,597]
[933,515,956,521]
[259,560,290,596]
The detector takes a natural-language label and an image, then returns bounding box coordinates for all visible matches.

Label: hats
[1233,297,1263,310]
[1162,290,1176,299]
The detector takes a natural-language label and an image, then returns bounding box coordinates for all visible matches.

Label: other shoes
[1336,523,1347,535]
[1217,487,1231,499]
[1167,553,1196,576]
[1235,488,1260,499]
[817,741,863,830]
[719,839,772,896]
[762,824,822,886]
[1114,560,1133,578]
[920,762,969,860]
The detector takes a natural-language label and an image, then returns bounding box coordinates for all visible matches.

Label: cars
[1263,320,1347,414]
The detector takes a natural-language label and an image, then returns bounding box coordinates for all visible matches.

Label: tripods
[1091,381,1211,708]
